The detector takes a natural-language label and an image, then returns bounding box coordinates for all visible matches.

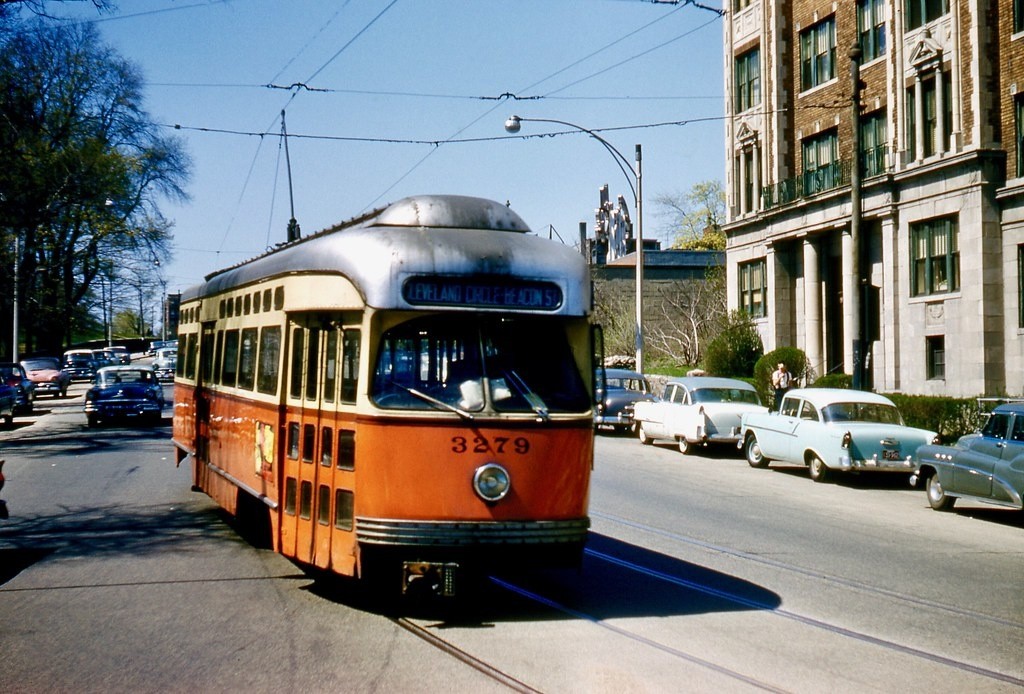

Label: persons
[772,363,793,409]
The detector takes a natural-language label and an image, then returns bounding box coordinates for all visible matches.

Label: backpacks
[770,370,789,389]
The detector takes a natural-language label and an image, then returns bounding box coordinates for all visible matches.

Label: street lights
[504,116,642,376]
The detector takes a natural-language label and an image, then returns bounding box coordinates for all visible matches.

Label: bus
[170,194,596,579]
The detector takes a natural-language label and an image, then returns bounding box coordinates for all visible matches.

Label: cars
[627,376,764,454]
[93,349,120,369]
[166,339,177,349]
[62,350,99,379]
[158,348,177,364]
[730,387,942,483]
[6,363,35,414]
[82,366,163,426]
[910,400,1024,514]
[22,358,71,398]
[595,369,660,434]
[153,360,175,384]
[0,369,22,422]
[148,342,166,356]
[105,346,130,366]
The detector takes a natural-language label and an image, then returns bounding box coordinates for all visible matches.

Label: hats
[778,360,785,369]
